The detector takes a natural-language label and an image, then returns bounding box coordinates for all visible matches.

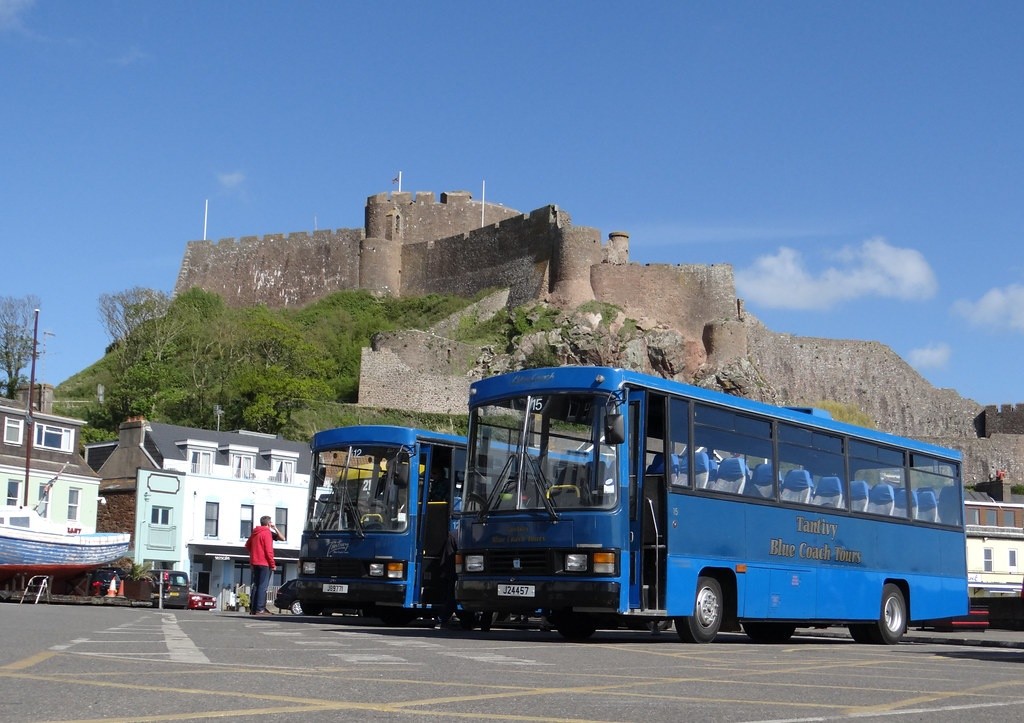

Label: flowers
[237,591,249,607]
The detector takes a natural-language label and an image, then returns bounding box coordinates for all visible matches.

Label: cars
[94,570,122,593]
[272,577,364,617]
[187,587,217,611]
[103,568,127,581]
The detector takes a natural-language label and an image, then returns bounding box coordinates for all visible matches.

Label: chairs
[557,451,959,527]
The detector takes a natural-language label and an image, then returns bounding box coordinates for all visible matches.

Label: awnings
[187,544,300,562]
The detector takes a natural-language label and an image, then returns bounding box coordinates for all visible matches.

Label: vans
[146,568,189,607]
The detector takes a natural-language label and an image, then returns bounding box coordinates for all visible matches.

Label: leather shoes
[249,608,257,615]
[255,608,274,615]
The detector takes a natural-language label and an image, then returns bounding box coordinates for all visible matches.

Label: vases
[239,606,246,612]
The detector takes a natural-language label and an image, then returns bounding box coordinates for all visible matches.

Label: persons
[245,516,286,616]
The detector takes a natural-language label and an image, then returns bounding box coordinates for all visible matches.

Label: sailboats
[0,306,134,574]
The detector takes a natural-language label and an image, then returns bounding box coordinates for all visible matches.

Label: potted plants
[124,560,157,603]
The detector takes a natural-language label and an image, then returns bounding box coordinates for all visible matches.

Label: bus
[296,425,618,627]
[936,501,1024,604]
[448,365,970,645]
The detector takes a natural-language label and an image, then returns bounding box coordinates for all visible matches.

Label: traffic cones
[104,577,118,598]
[116,580,127,600]
